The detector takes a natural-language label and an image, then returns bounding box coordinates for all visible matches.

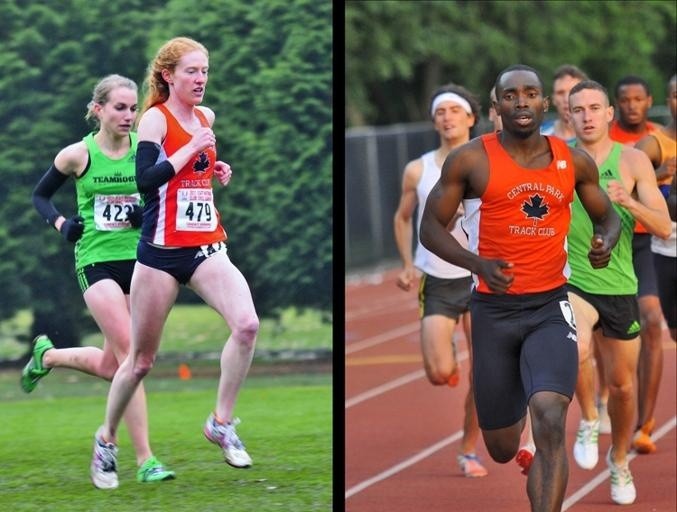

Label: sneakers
[21,334,54,393]
[89,425,119,490]
[455,454,487,478]
[136,456,176,481]
[516,397,657,506]
[202,411,253,467]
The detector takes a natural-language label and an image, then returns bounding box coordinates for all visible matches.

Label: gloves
[128,205,144,227]
[61,215,84,244]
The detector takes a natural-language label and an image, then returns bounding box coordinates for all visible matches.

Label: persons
[90,37,261,490]
[392,65,677,510]
[19,73,176,483]
